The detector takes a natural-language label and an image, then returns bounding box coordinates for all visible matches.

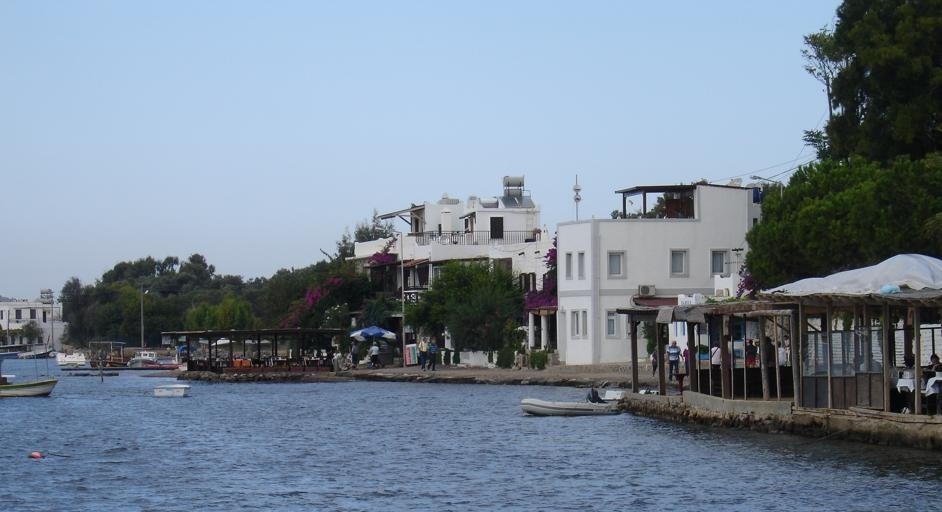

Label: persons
[419,337,428,371]
[860,353,942,414]
[711,341,721,387]
[666,341,684,381]
[428,336,437,371]
[368,341,378,368]
[746,335,790,368]
[683,342,689,377]
[651,344,658,378]
[351,338,359,369]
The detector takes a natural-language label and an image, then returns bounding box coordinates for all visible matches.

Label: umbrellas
[350,325,396,343]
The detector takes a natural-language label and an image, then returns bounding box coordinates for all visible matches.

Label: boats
[0,376,57,397]
[600,390,660,402]
[56,351,85,367]
[89,342,126,367]
[127,351,179,370]
[521,397,620,415]
[0,344,53,359]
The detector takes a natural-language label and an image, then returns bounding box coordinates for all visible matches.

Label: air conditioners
[715,289,728,297]
[638,284,656,296]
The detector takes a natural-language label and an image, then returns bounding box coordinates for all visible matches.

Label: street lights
[375,227,406,368]
[128,277,144,347]
[750,175,783,202]
[41,288,54,352]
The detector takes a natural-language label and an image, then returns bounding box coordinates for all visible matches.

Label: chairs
[888,365,942,416]
[189,352,335,370]
